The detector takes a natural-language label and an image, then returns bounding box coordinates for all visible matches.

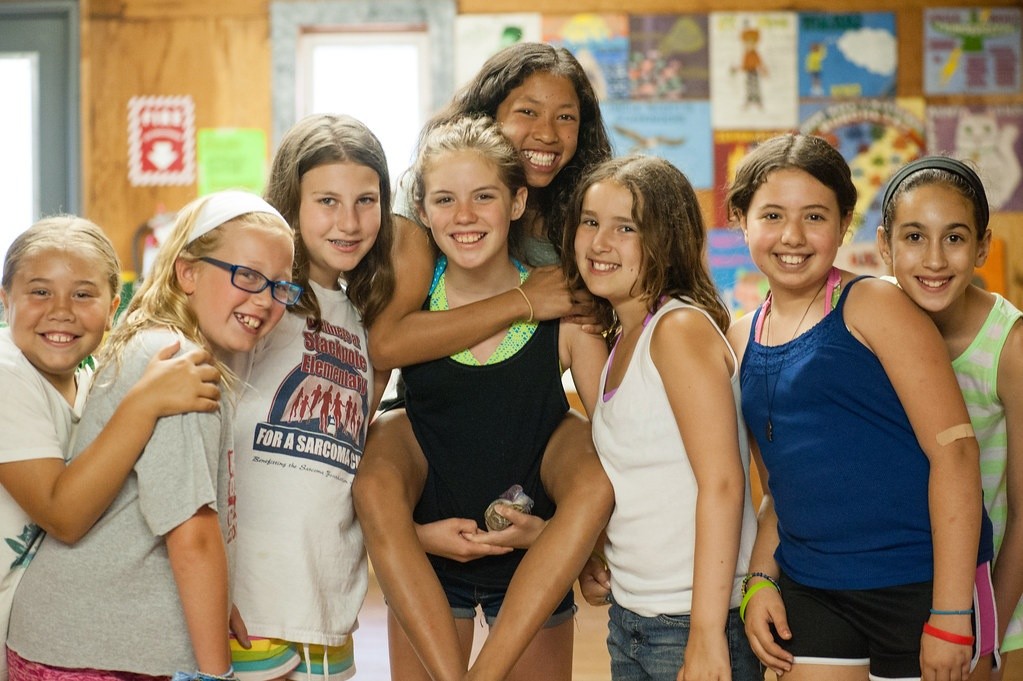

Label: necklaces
[764,278,828,442]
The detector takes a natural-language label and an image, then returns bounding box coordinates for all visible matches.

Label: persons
[721,133,1001,681]
[877,156,1023,681]
[368,116,608,681]
[0,217,221,680]
[228,125,394,681]
[352,44,614,680]
[8,189,302,681]
[561,155,765,681]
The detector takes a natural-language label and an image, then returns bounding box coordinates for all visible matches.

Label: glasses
[199,255,302,306]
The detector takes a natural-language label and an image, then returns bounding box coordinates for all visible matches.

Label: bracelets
[585,548,611,574]
[175,664,237,681]
[739,572,782,622]
[513,285,534,324]
[925,606,975,617]
[920,621,976,645]
